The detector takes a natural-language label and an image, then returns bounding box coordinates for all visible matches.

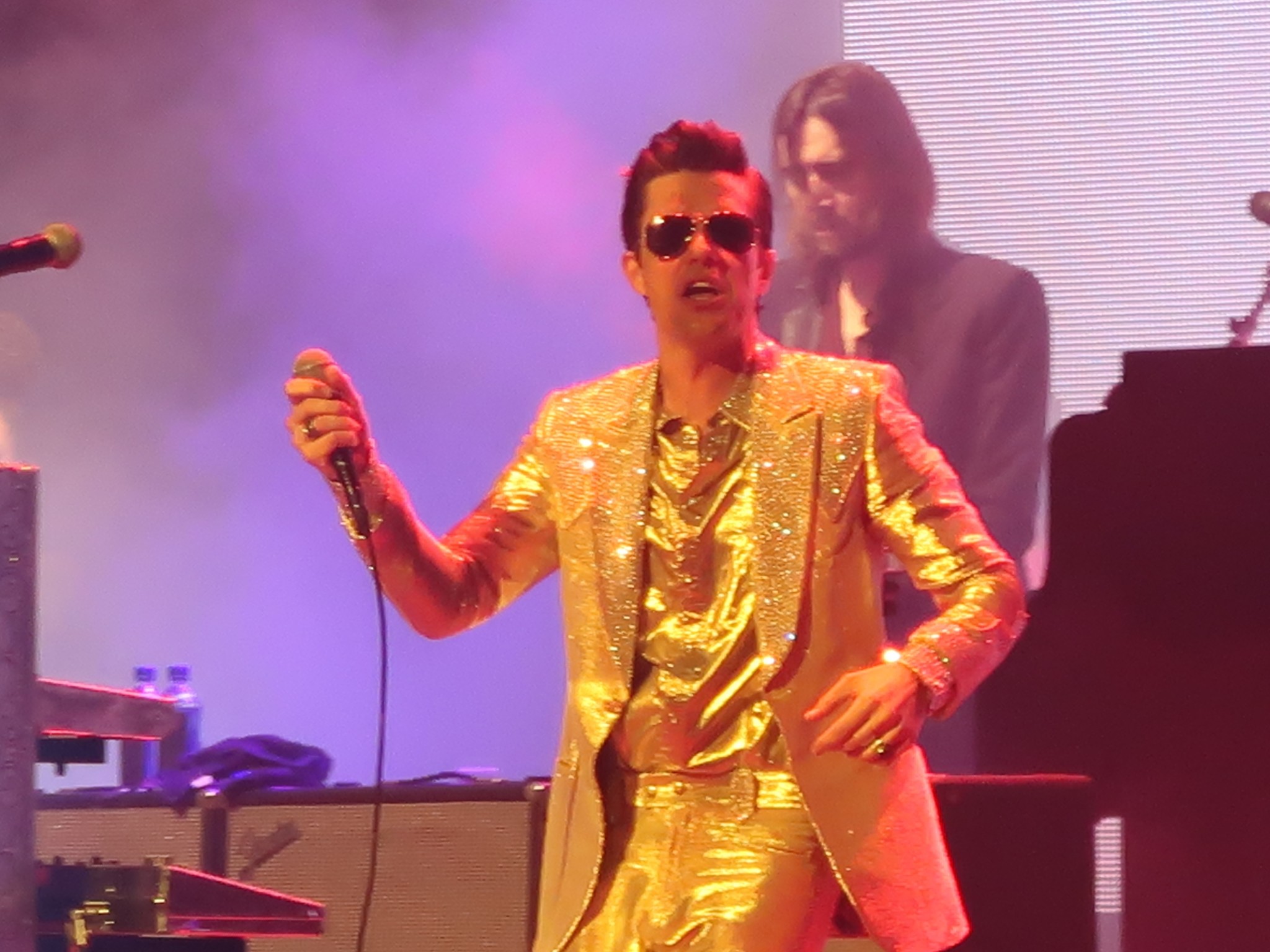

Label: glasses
[637,213,763,262]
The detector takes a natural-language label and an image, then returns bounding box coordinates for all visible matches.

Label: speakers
[33,780,552,952]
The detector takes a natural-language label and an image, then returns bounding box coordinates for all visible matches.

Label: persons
[755,61,1050,774]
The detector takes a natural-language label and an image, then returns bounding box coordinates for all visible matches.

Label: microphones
[1250,191,1270,224]
[291,349,370,535]
[0,222,84,277]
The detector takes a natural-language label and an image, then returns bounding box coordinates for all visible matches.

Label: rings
[283,119,1030,951]
[300,414,322,439]
[870,739,893,758]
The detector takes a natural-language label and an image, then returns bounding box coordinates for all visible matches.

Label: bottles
[160,666,199,783]
[121,666,161,786]
